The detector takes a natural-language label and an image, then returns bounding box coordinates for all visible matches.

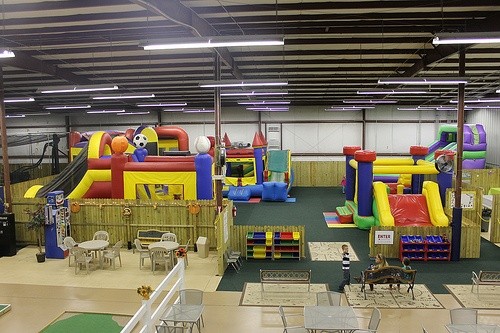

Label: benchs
[471,270,500,296]
[136,230,170,245]
[260,269,311,299]
[360,265,417,300]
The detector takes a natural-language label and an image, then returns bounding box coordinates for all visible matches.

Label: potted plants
[23,198,47,263]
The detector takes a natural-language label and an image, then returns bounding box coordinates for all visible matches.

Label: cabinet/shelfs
[399,234,451,264]
[246,231,302,261]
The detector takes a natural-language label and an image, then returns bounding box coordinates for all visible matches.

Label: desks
[78,240,110,269]
[304,306,360,333]
[148,240,179,268]
[159,304,205,333]
[444,323,500,333]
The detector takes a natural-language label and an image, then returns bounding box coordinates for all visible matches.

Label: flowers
[176,247,187,258]
[137,285,153,299]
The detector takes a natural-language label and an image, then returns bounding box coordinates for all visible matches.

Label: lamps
[0,0,500,118]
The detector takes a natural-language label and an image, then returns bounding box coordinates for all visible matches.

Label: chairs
[63,230,123,274]
[278,291,381,333]
[223,246,243,273]
[134,233,192,275]
[155,288,204,333]
[450,307,478,324]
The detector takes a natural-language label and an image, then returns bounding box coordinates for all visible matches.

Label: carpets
[344,282,446,309]
[442,283,500,310]
[239,281,333,307]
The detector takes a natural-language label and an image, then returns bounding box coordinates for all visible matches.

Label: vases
[141,296,148,300]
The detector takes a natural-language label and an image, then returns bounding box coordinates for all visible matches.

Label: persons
[341,177,348,193]
[393,258,412,280]
[339,245,350,293]
[353,254,388,282]
